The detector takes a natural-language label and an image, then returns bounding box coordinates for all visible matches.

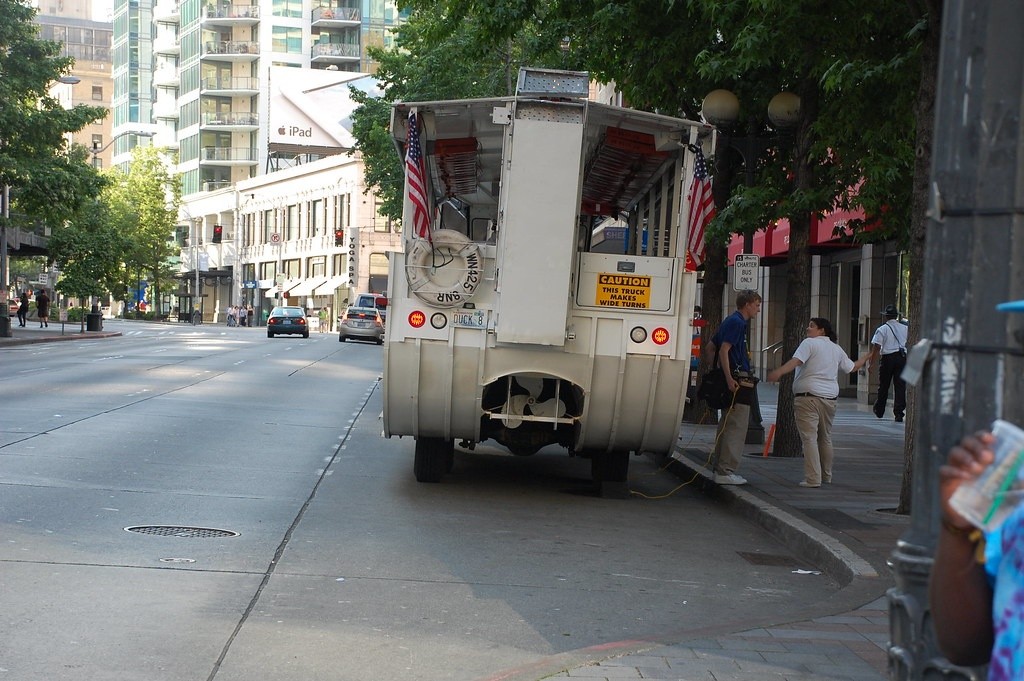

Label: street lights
[88,131,156,314]
[701,90,803,366]
[163,203,193,315]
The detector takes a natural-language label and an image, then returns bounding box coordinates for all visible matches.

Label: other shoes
[20,324,25,327]
[822,478,831,485]
[799,480,821,487]
[40,325,43,328]
[45,322,48,327]
[895,415,903,422]
[873,410,883,419]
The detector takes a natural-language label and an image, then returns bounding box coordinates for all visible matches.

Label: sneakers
[712,471,747,484]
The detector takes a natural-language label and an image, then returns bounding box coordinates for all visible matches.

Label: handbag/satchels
[900,349,907,358]
[697,371,734,408]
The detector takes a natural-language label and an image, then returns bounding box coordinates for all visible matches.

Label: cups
[947,419,1024,530]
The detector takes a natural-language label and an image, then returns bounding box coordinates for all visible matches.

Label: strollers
[227,315,239,327]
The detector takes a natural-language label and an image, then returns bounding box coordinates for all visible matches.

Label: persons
[226,304,253,327]
[319,307,329,334]
[36,289,50,328]
[139,300,148,314]
[17,293,29,327]
[175,301,179,320]
[768,317,874,486]
[931,300,1023,681]
[705,289,762,484]
[867,305,908,422]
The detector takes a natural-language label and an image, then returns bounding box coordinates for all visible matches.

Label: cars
[338,306,385,345]
[10,297,35,318]
[266,305,311,338]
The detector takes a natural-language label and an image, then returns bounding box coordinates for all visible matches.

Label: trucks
[353,293,386,330]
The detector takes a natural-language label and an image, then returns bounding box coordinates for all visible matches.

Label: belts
[795,392,837,400]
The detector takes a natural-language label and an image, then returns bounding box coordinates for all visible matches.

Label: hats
[995,300,1024,314]
[880,305,903,316]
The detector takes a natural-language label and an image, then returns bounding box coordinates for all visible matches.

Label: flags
[684,144,713,269]
[405,111,433,242]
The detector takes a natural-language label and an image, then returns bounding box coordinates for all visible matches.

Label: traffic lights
[212,225,223,243]
[283,292,290,298]
[334,230,344,247]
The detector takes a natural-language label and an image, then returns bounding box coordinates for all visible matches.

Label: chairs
[360,299,368,306]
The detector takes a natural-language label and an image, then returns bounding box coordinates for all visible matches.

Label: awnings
[265,278,348,298]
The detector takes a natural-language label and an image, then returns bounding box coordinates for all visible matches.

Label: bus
[376,63,719,483]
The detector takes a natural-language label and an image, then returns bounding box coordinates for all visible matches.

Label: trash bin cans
[248,310,253,328]
[87,313,103,331]
[0,316,12,338]
[682,365,699,423]
[101,307,111,318]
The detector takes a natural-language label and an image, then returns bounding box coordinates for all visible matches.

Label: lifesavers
[407,229,482,306]
